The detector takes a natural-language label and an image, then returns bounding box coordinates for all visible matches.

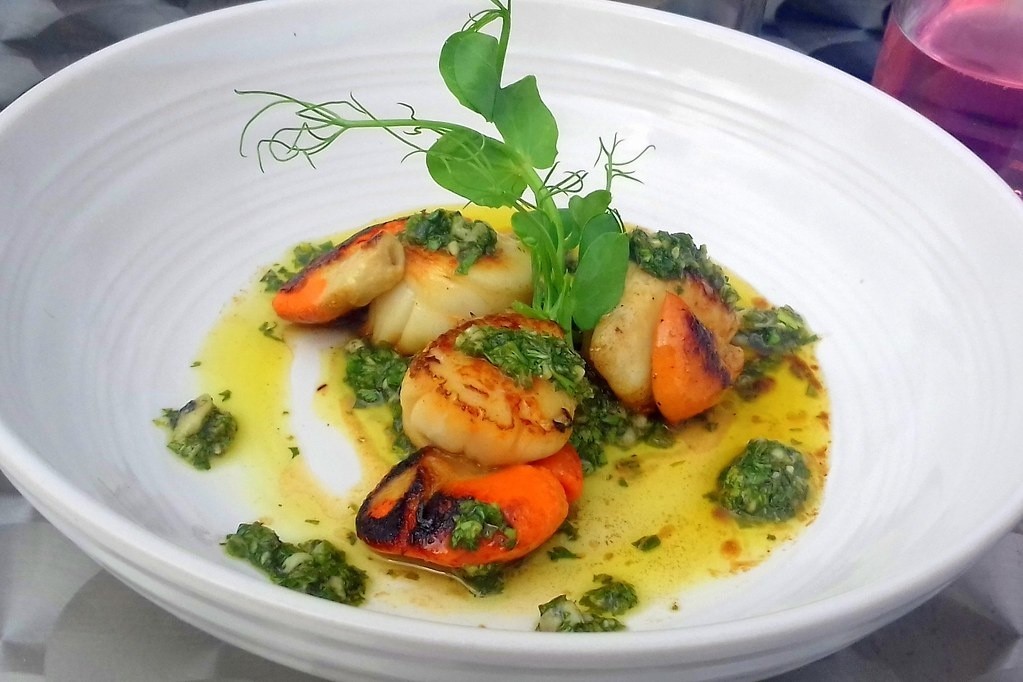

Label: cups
[869,0,1023,200]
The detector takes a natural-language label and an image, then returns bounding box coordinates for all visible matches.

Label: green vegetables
[154,0,824,638]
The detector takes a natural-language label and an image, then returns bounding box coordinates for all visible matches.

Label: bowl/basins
[0,0,1023,682]
